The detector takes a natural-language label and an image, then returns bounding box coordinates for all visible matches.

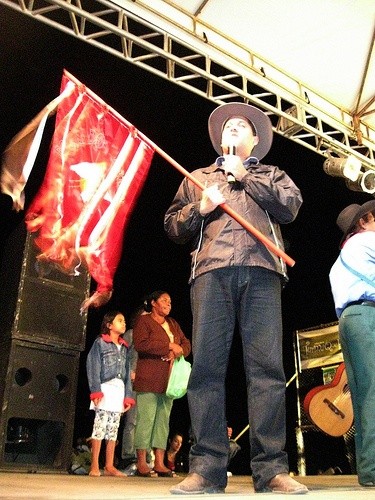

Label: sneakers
[170,472,225,494]
[255,473,308,494]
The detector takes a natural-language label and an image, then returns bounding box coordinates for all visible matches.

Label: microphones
[227,146,235,184]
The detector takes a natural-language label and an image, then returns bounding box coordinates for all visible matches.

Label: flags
[0,68,154,316]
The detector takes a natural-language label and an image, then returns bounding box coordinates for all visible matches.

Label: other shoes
[103,466,127,477]
[136,468,158,478]
[89,471,101,477]
[152,468,177,478]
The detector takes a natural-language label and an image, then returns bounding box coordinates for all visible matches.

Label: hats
[337,200,375,250]
[208,102,273,161]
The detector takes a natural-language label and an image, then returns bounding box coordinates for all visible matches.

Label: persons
[163,101,307,494]
[189,418,241,477]
[86,291,191,477]
[329,201,375,487]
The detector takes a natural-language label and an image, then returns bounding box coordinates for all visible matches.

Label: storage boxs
[0,230,92,352]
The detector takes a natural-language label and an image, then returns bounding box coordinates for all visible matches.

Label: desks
[0,339,81,475]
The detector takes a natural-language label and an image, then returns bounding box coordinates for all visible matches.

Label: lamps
[322,155,363,181]
[345,170,375,194]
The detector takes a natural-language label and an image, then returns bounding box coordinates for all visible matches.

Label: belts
[340,299,375,316]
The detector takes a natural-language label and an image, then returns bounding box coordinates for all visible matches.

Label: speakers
[0,212,90,473]
[291,321,356,476]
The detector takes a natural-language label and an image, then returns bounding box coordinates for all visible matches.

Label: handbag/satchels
[67,450,92,475]
[165,354,192,399]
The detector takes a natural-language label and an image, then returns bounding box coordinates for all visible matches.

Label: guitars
[303,361,354,437]
